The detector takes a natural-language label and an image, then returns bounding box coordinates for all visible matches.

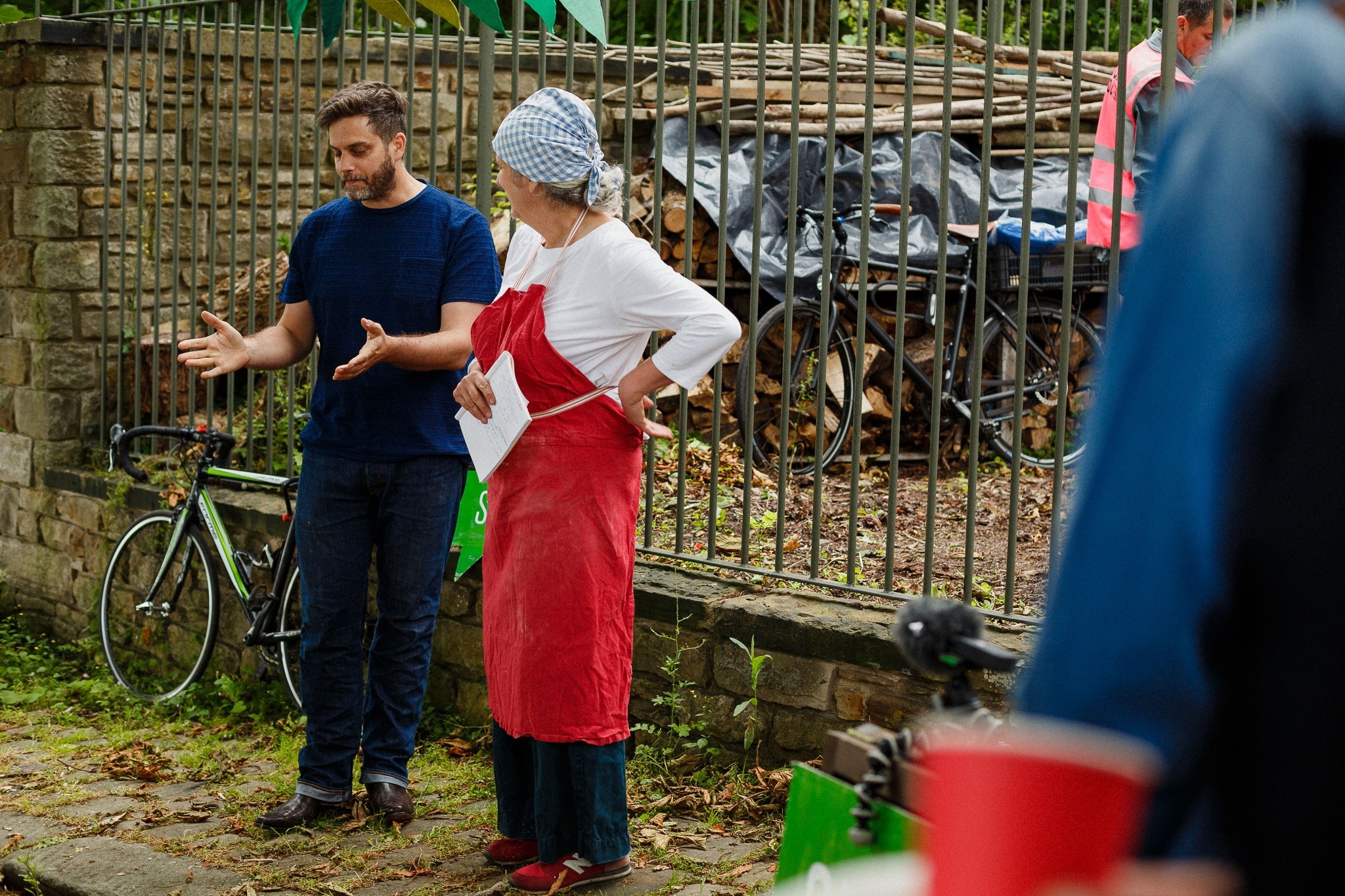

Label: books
[455,351,531,483]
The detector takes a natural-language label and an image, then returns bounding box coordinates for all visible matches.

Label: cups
[911,711,1164,896]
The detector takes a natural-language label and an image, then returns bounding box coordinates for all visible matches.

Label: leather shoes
[255,793,321,829]
[364,781,415,822]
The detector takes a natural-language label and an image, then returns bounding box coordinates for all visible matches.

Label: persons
[1086,0,1233,270]
[452,86,740,892]
[175,80,502,826]
[1009,0,1345,896]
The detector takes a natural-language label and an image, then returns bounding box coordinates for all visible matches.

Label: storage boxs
[974,244,1110,289]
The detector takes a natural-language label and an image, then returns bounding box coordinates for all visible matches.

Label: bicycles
[728,200,1113,483]
[98,420,383,712]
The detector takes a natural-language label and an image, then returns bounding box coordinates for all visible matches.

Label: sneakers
[509,848,632,893]
[483,836,539,865]
